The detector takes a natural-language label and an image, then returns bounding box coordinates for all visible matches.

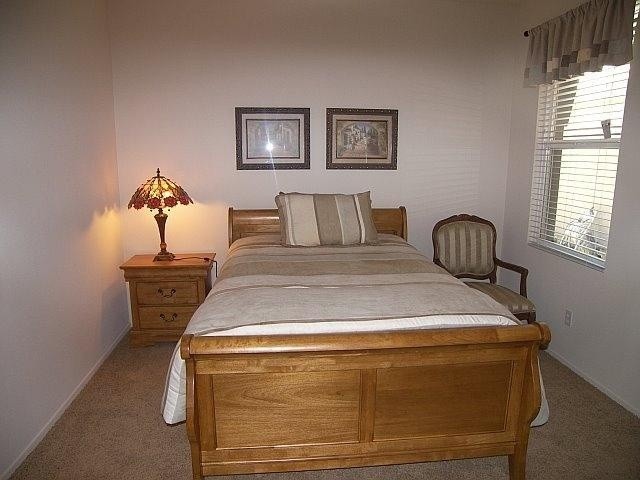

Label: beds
[159,205,552,480]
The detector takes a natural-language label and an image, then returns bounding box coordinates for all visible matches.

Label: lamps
[127,167,217,278]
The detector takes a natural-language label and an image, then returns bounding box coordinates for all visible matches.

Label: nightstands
[119,252,216,349]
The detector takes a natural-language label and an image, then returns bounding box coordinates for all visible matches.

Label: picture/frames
[326,107,398,170]
[235,107,310,171]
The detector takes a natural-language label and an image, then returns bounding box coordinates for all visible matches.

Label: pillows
[275,190,385,247]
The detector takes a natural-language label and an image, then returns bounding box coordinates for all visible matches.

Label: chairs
[432,213,537,324]
